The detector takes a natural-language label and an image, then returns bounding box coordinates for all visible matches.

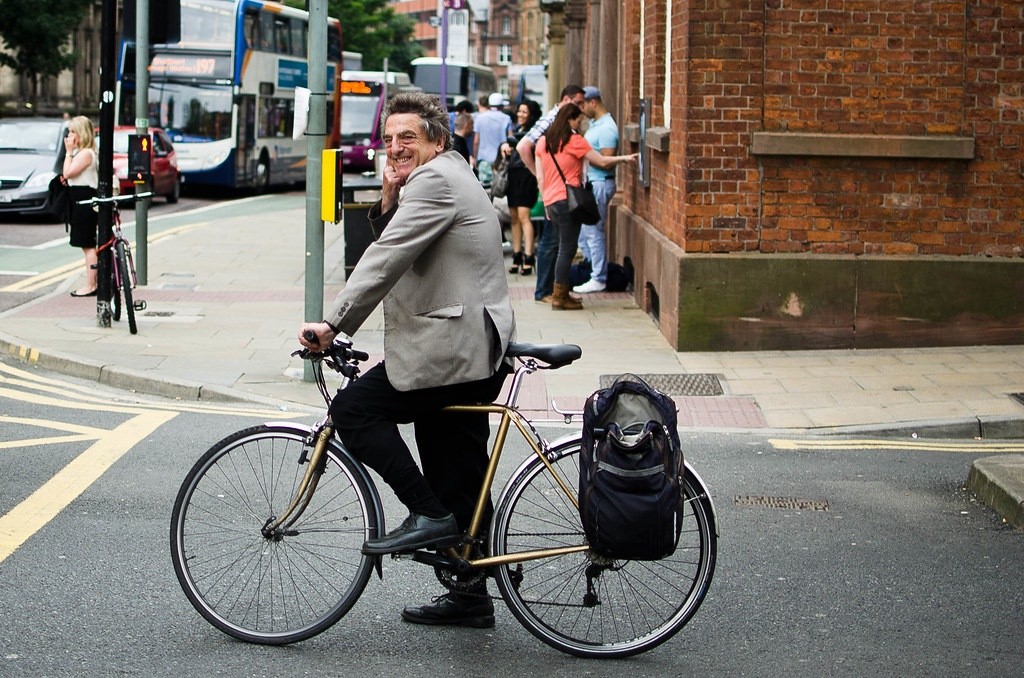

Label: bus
[342,57,548,174]
[114,0,343,196]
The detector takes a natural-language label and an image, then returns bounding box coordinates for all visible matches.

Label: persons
[535,104,640,311]
[60,116,99,296]
[498,101,541,276]
[572,86,619,293]
[451,92,514,182]
[515,85,586,303]
[298,92,517,628]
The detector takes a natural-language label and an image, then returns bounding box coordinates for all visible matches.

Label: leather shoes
[361,512,458,556]
[400,592,495,628]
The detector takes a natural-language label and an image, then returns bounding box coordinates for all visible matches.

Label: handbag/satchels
[491,165,509,198]
[578,372,684,561]
[566,183,601,225]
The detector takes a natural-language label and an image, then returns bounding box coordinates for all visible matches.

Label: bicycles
[169,330,720,658]
[76,191,156,334]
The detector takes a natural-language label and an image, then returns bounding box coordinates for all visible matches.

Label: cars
[0,118,71,223]
[92,127,180,208]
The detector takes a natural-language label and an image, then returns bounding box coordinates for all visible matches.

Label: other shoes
[70,288,97,296]
[535,294,552,305]
[572,279,606,293]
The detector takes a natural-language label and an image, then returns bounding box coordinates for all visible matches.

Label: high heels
[509,251,523,273]
[521,253,536,275]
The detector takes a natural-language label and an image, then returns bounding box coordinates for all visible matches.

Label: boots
[551,281,583,310]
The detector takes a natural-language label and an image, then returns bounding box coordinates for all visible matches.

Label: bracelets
[65,154,73,157]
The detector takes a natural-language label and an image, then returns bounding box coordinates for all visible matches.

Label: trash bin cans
[342,171,383,283]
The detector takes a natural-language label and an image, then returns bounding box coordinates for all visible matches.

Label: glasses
[571,97,585,108]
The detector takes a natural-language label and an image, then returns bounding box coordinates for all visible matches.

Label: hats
[489,92,510,106]
[581,86,600,99]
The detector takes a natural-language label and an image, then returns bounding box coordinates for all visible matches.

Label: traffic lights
[321,149,344,225]
[127,134,152,184]
